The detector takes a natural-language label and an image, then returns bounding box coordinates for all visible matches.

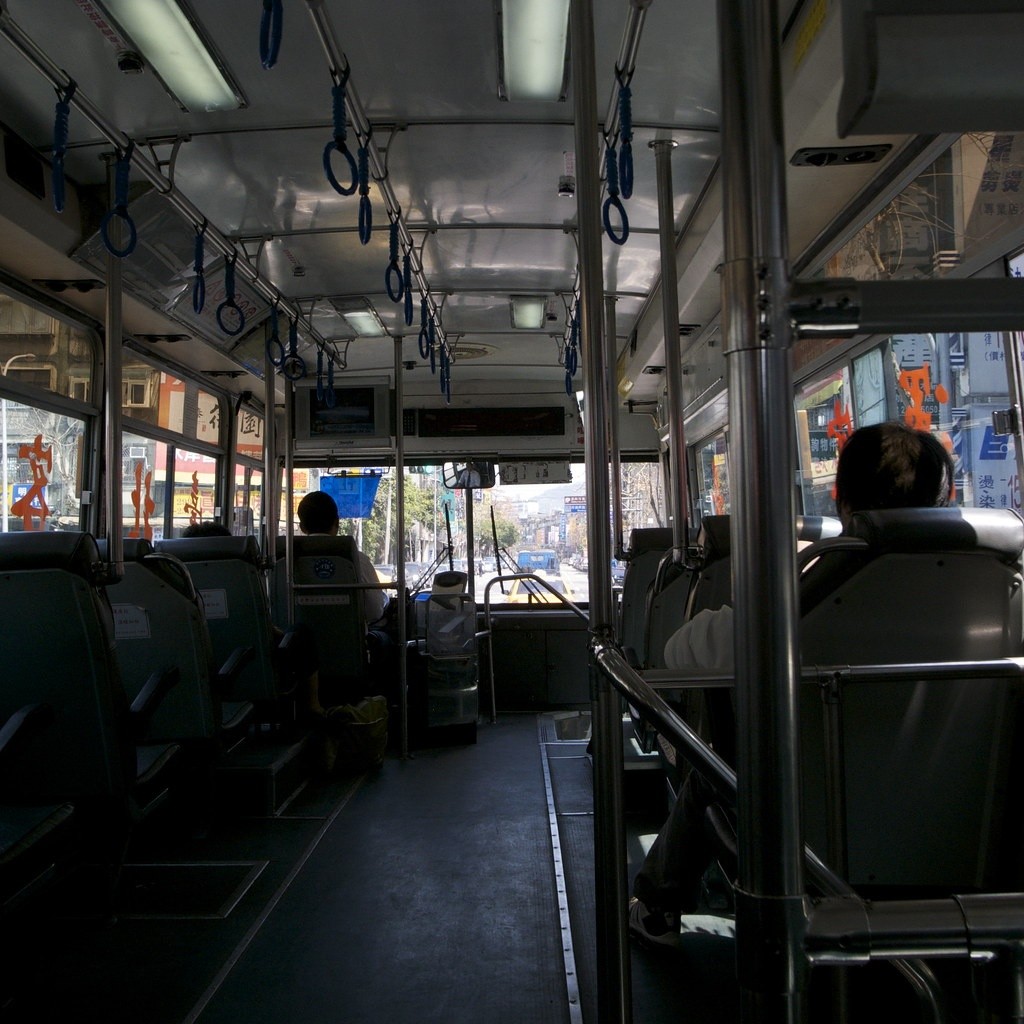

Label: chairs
[623,525,1023,1024]
[1,527,374,926]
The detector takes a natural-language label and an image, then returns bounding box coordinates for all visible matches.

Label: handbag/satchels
[326,694,390,750]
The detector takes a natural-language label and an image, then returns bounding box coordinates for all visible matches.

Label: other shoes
[627,894,683,950]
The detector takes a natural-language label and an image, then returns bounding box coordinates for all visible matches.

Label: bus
[517,549,563,590]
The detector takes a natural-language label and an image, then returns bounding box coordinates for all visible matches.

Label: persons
[265,491,403,713]
[181,521,323,709]
[457,462,481,488]
[625,419,959,946]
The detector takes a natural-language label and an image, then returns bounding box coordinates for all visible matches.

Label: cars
[372,554,511,595]
[567,555,626,585]
[502,576,576,603]
